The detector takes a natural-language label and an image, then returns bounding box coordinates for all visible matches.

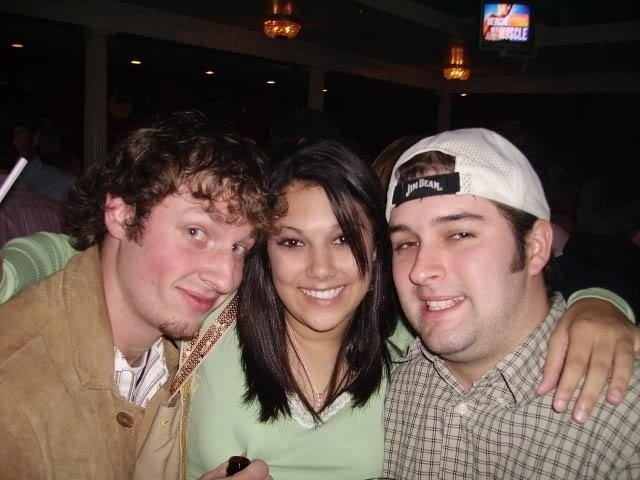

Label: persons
[1,111,287,480]
[0,138,639,480]
[370,133,423,198]
[379,125,638,478]
[1,113,95,247]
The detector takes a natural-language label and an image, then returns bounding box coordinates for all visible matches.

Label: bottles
[226,455,250,477]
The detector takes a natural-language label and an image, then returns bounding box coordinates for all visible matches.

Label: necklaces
[286,323,353,407]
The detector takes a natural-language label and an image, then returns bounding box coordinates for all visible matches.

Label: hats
[384,125,551,222]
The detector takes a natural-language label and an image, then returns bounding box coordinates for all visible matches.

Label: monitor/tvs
[477,0,535,52]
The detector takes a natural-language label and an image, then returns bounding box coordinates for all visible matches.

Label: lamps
[441,44,476,82]
[260,0,305,43]
